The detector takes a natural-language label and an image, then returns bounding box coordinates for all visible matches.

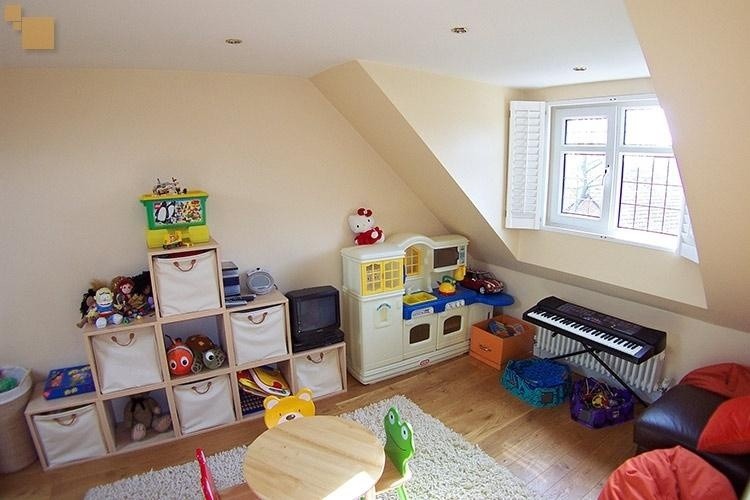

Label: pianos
[522,295,666,365]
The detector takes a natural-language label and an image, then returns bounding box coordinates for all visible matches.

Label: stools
[635,380,749,491]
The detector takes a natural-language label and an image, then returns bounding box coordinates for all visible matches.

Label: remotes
[226,300,247,305]
[225,294,256,301]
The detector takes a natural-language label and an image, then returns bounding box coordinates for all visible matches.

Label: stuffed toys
[78,268,155,329]
[166,332,193,376]
[122,392,172,443]
[347,206,388,244]
[184,334,227,375]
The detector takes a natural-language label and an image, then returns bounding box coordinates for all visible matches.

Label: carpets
[86,394,546,500]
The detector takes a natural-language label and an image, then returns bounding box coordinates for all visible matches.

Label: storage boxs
[228,303,289,366]
[32,403,109,467]
[139,191,209,229]
[171,374,238,435]
[469,313,537,371]
[88,325,165,395]
[151,250,224,315]
[293,346,342,399]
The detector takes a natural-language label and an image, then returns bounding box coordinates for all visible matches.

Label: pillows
[696,396,750,457]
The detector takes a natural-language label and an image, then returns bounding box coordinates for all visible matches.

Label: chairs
[195,387,416,500]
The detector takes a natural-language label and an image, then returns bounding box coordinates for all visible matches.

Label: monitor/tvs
[284,285,346,353]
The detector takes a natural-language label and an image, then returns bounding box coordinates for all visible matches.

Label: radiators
[537,326,665,404]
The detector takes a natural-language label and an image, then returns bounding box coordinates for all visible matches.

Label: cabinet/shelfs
[24,238,348,472]
